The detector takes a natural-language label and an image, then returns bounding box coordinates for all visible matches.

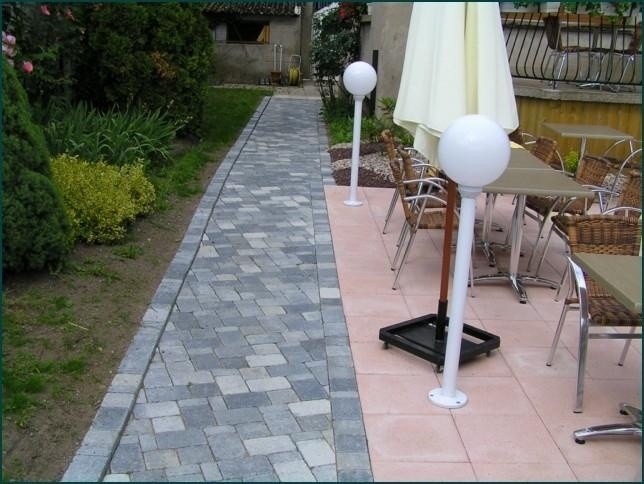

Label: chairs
[541,15,643,94]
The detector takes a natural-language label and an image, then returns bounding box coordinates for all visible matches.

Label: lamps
[342,60,377,206]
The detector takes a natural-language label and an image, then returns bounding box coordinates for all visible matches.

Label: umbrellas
[391,0,519,339]
